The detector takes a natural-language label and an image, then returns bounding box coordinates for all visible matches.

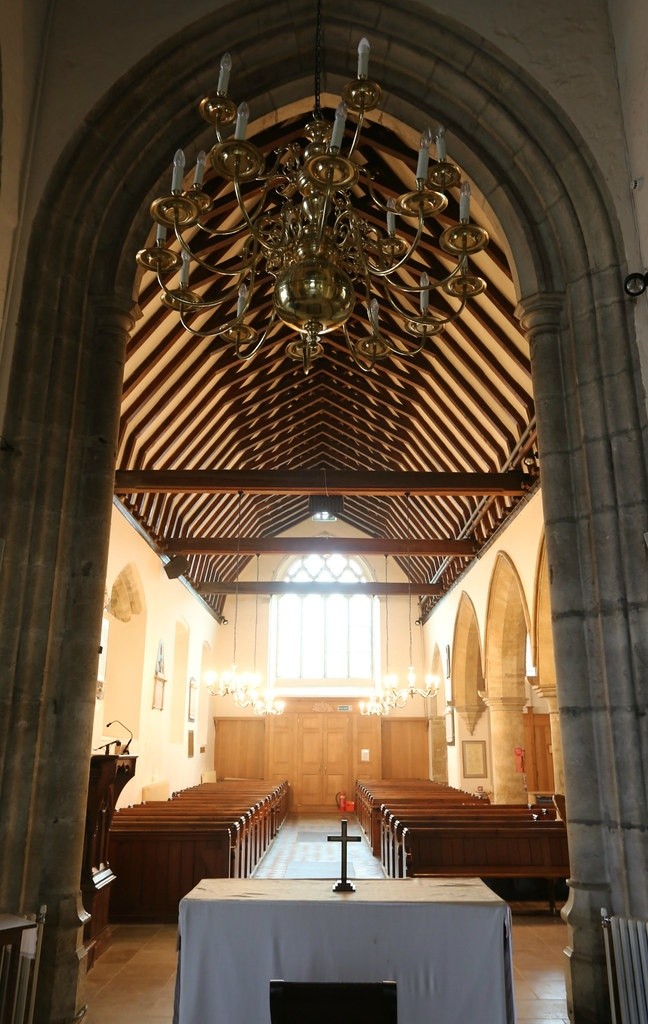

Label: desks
[172,878,515,1024]
[0,912,37,1024]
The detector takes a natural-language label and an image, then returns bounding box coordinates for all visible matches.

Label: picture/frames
[445,710,455,745]
[152,676,167,711]
[189,682,199,721]
[461,740,487,778]
[188,730,195,758]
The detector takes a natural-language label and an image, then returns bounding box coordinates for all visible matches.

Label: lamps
[136,0,490,375]
[202,491,285,715]
[359,492,439,715]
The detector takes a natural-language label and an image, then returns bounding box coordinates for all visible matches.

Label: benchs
[103,778,571,925]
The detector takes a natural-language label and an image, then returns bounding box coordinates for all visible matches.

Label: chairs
[268,978,398,1024]
